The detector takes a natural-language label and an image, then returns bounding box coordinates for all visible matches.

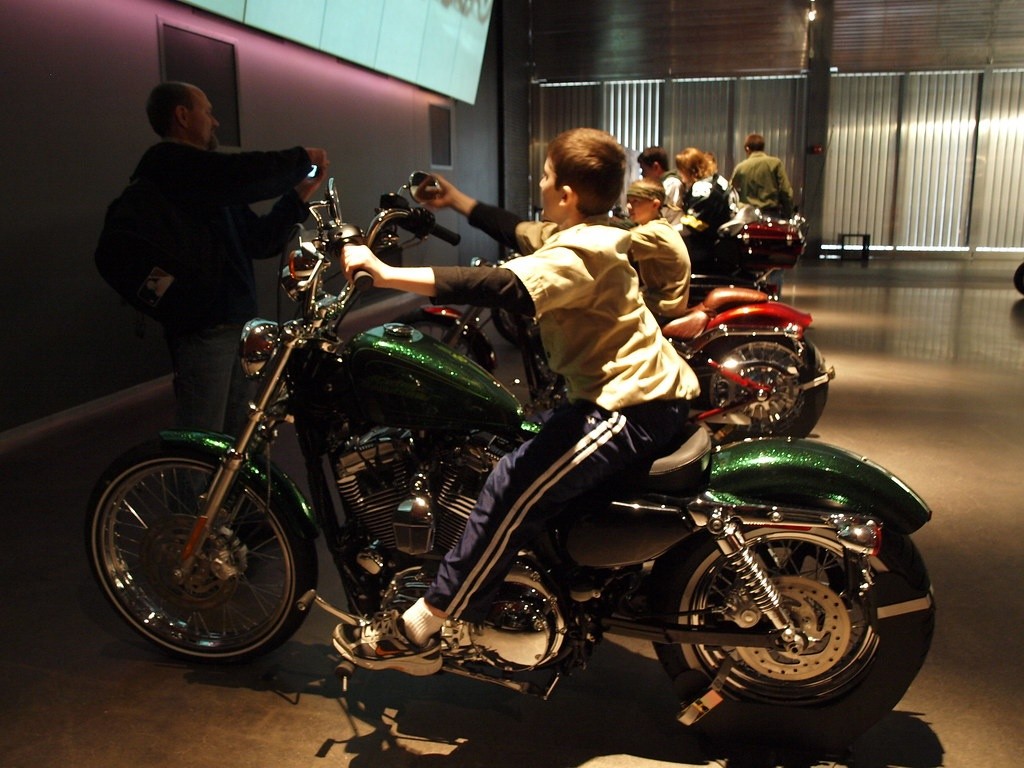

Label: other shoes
[233,513,274,540]
[239,554,259,580]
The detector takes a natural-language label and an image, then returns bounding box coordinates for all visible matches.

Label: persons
[332,127,701,677]
[93,80,329,515]
[638,144,739,274]
[730,134,796,300]
[620,178,691,322]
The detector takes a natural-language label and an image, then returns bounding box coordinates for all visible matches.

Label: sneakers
[332,609,446,677]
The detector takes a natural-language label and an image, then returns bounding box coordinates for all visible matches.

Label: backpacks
[93,148,183,315]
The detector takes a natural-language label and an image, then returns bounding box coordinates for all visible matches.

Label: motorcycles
[84,170,936,766]
